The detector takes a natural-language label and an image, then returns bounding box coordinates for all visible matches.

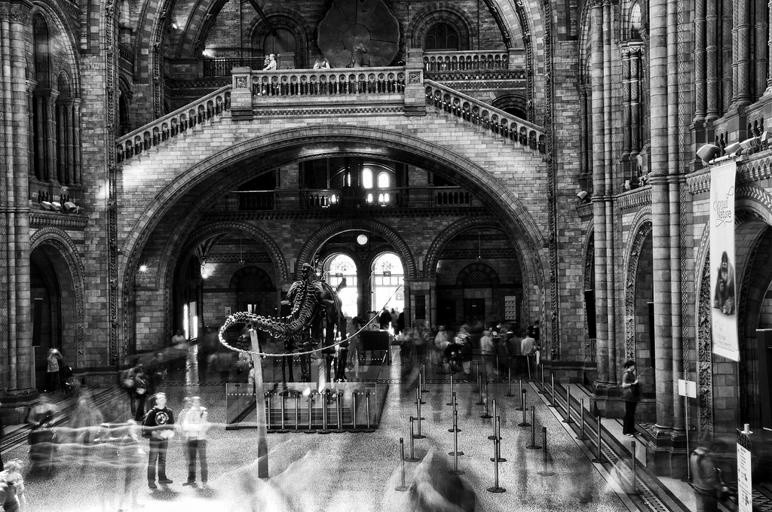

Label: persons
[688,438,728,512]
[96,419,148,512]
[619,360,645,436]
[0,458,27,512]
[261,53,280,72]
[376,306,548,377]
[26,328,217,489]
[311,51,332,71]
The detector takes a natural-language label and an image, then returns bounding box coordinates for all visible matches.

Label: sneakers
[145,478,197,490]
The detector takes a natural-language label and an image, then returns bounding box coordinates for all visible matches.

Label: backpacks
[142,410,154,437]
